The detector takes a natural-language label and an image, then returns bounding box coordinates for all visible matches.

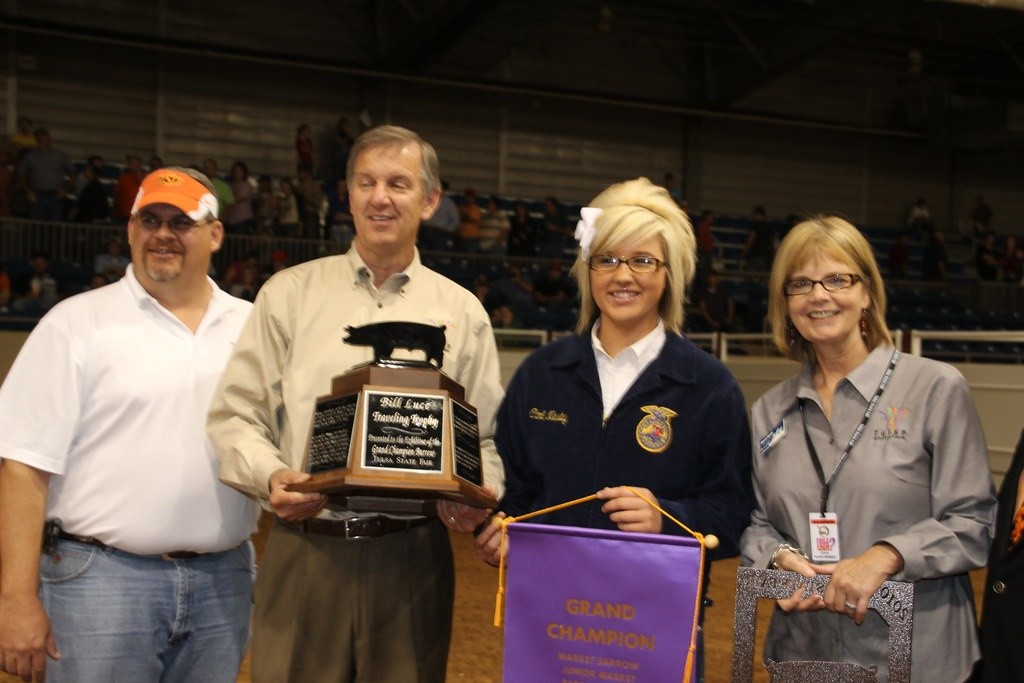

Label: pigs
[343,321,447,368]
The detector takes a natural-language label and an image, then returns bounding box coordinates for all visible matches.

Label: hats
[131,168,219,221]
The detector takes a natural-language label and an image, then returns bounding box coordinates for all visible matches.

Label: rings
[844,600,857,609]
[447,516,456,524]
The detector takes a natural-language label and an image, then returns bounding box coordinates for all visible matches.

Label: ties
[1007,500,1024,556]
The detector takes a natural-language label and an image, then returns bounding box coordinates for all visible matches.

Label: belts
[280,515,438,542]
[58,528,210,562]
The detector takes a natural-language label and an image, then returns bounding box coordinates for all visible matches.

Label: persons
[739,215,998,683]
[0,167,279,683]
[0,118,1024,356]
[473,175,755,683]
[206,125,506,683]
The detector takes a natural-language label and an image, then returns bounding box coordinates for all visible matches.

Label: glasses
[782,273,862,295]
[131,212,209,234]
[583,254,667,274]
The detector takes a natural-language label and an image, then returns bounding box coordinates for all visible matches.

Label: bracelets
[771,543,810,570]
